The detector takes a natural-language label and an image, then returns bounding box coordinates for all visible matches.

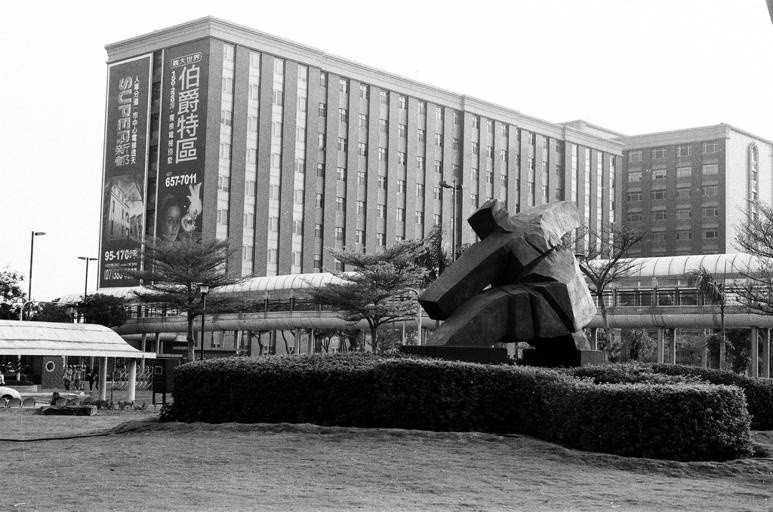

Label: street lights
[77,256,97,323]
[27,231,46,317]
[438,179,462,261]
[196,282,212,361]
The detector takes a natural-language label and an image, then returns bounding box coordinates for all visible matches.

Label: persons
[93,372,98,390]
[0,370,5,386]
[156,195,181,246]
[86,369,95,392]
[72,366,85,389]
[63,365,72,390]
